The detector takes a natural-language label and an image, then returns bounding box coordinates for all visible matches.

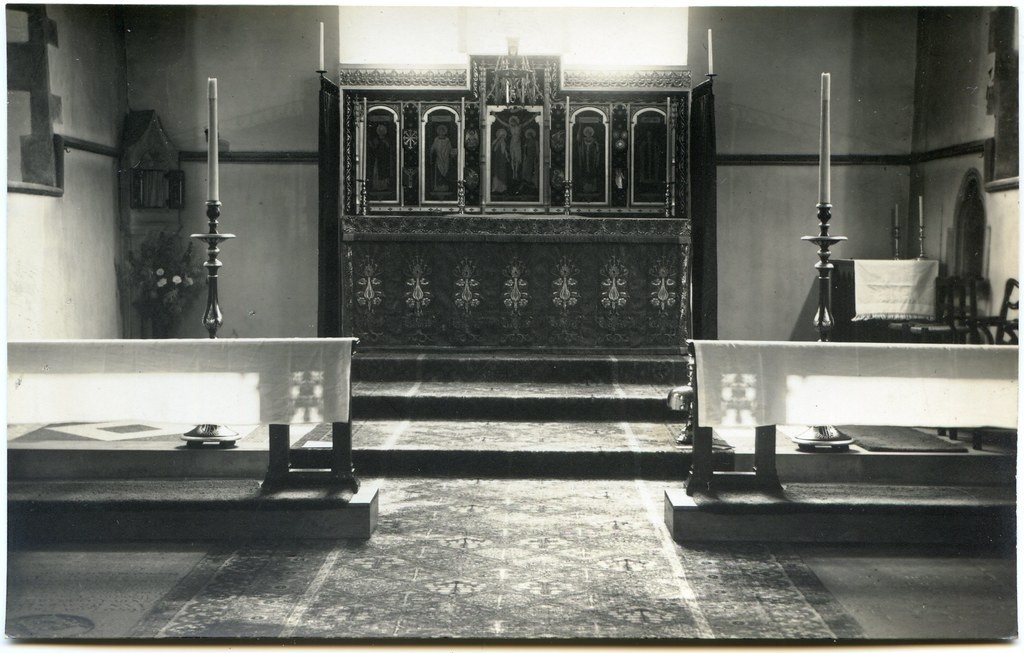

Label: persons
[372,124,391,190]
[580,127,600,193]
[430,125,452,186]
[639,129,663,184]
[491,113,540,193]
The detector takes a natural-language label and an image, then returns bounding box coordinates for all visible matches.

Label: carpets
[843,427,969,452]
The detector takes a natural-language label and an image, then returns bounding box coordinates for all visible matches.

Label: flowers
[126,224,207,333]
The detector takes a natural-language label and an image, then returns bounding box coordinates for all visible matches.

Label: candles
[460,97,464,180]
[919,196,924,227]
[207,78,219,201]
[362,97,366,179]
[565,95,570,180]
[319,22,325,69]
[895,204,898,228]
[819,72,832,204]
[666,97,670,183]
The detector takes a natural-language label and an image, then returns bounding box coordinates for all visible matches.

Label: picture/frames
[983,6,1019,193]
